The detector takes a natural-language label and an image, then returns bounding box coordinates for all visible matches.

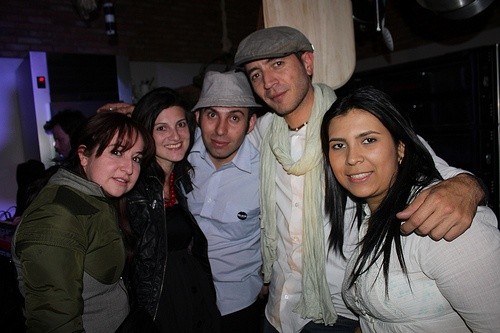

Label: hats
[192,71,262,114]
[234,26,314,69]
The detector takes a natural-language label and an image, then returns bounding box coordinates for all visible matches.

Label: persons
[12,106,147,332]
[124,84,222,332]
[178,69,279,332]
[321,86,499,333]
[235,24,485,333]
[41,105,89,163]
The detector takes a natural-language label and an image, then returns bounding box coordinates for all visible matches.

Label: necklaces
[160,173,176,208]
[287,122,309,132]
